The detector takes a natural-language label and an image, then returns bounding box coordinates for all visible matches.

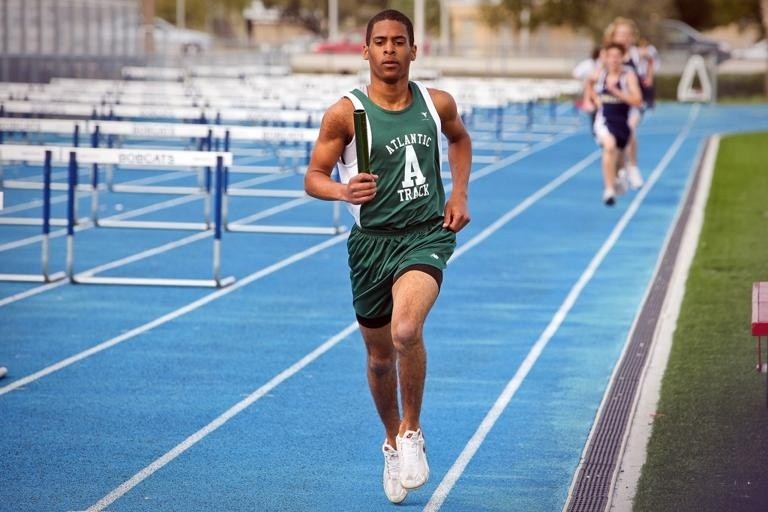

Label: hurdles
[0,64,587,287]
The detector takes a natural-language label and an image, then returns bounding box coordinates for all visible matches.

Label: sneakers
[381,438,409,504]
[395,427,429,489]
[602,165,642,205]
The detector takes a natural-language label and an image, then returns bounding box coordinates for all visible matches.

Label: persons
[570,12,659,208]
[302,10,472,505]
